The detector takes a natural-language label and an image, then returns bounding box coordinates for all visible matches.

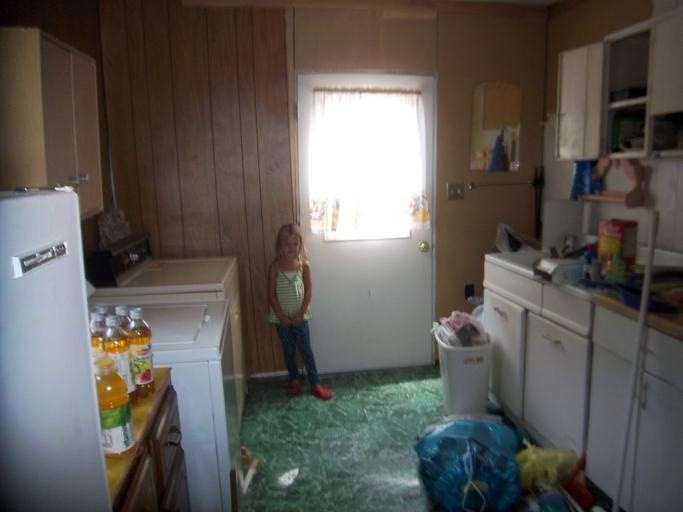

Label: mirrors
[468,81,522,173]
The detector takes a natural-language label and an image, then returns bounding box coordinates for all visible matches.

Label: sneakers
[310,385,332,399]
[286,382,298,395]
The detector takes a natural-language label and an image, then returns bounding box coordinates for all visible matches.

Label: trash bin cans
[433,326,494,415]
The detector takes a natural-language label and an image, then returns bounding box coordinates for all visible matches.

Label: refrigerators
[0,184,109,512]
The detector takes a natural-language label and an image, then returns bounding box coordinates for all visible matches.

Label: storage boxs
[598,218,638,273]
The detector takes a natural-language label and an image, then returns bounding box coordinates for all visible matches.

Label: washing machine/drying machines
[115,253,250,422]
[86,293,241,512]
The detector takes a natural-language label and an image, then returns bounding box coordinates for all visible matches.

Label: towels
[488,130,507,171]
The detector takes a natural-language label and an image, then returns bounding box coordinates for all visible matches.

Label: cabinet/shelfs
[602,7,683,160]
[482,262,591,471]
[0,25,105,222]
[583,305,683,512]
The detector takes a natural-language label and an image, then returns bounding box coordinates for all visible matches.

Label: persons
[266,224,332,400]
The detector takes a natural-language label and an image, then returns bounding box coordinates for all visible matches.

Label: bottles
[90,303,152,461]
[537,478,567,512]
[582,244,625,286]
